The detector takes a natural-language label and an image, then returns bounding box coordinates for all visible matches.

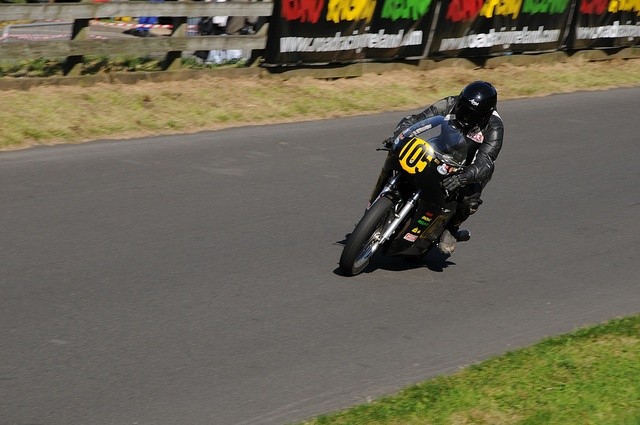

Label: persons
[370,79,504,256]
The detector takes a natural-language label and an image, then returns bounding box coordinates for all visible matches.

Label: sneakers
[439,222,460,256]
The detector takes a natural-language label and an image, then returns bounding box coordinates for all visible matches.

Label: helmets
[455,81,497,129]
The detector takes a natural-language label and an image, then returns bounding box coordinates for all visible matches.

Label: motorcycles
[339,115,471,277]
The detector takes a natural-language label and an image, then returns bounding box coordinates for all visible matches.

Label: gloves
[394,125,410,140]
[440,170,474,197]
